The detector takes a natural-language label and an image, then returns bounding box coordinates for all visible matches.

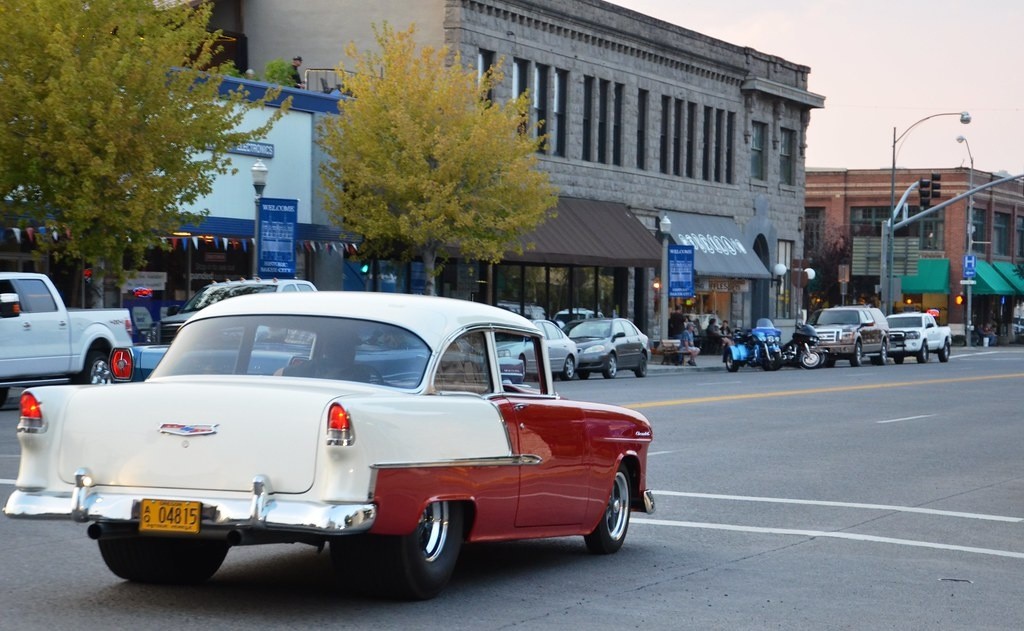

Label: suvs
[813,307,890,367]
[156,279,319,347]
[497,300,546,320]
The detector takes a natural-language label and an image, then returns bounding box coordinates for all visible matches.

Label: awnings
[967,261,1024,295]
[896,258,951,294]
[657,210,772,279]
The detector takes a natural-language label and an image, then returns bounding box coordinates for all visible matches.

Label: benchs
[660,338,696,365]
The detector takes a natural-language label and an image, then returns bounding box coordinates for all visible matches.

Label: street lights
[889,111,971,312]
[656,214,671,350]
[251,159,269,278]
[955,136,972,346]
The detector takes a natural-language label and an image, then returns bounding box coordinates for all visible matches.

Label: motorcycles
[780,322,827,369]
[723,317,783,372]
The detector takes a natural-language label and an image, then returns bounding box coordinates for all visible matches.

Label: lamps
[768,263,786,288]
[803,267,816,281]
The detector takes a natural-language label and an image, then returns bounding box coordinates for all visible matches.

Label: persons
[272,327,371,376]
[978,316,998,347]
[669,305,735,367]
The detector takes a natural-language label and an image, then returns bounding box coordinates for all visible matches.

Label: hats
[687,322,696,327]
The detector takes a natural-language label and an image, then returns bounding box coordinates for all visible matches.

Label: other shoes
[688,359,696,366]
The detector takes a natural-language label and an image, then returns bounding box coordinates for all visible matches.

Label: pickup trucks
[0,270,136,408]
[885,312,952,365]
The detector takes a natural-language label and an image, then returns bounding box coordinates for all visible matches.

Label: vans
[554,306,602,323]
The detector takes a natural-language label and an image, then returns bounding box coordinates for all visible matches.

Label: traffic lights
[920,176,930,206]
[956,295,967,305]
[930,172,941,198]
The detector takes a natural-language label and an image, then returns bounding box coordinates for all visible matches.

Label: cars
[2,291,654,597]
[489,318,580,381]
[108,308,525,405]
[560,316,649,379]
